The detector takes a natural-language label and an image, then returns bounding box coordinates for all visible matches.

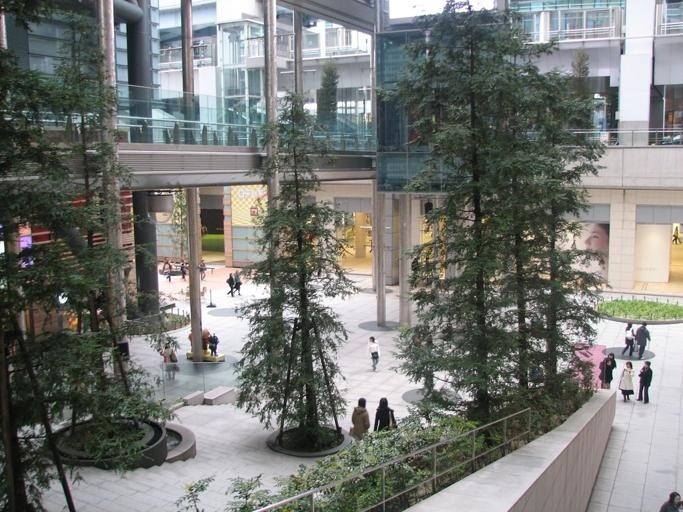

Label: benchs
[185,351,224,362]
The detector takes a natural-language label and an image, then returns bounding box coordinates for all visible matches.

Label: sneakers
[623,398,650,403]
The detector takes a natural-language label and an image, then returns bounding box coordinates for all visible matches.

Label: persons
[351,398,370,441]
[189,330,219,356]
[583,224,609,283]
[163,346,178,381]
[621,322,636,356]
[659,491,681,512]
[599,352,616,389]
[374,397,396,433]
[163,257,172,282]
[368,336,380,371]
[635,321,651,360]
[227,273,234,297]
[233,270,241,295]
[200,260,207,280]
[618,362,634,402]
[677,501,683,512]
[180,264,186,279]
[635,361,652,404]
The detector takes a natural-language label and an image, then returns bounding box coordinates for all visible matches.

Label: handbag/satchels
[634,343,638,352]
[371,351,379,359]
[170,352,178,362]
